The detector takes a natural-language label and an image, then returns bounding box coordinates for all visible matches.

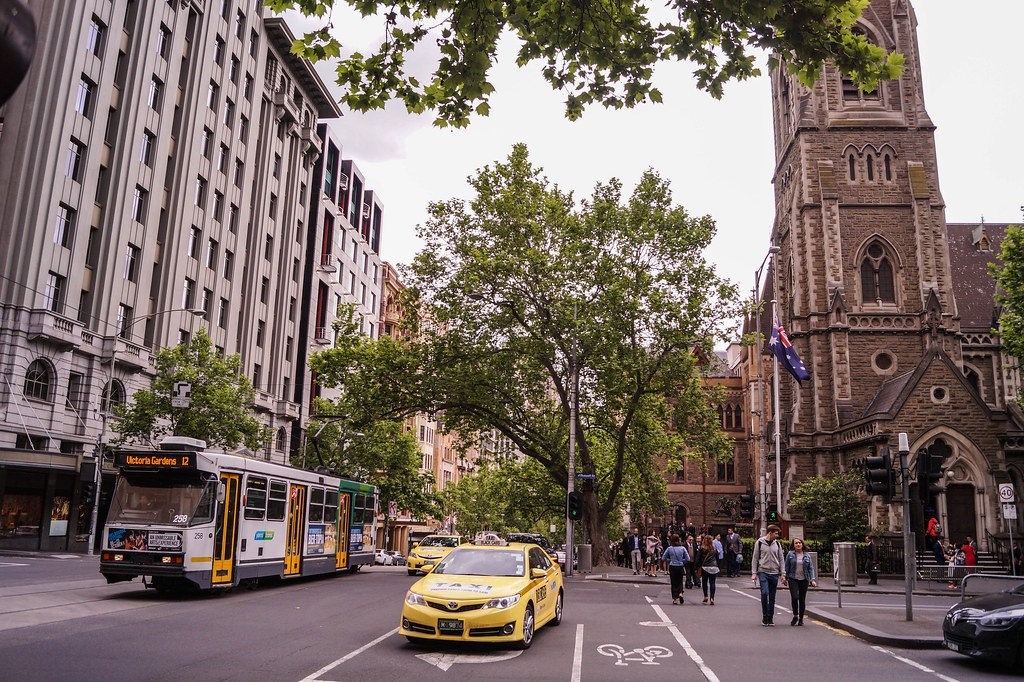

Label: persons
[122,529,148,551]
[933,536,948,583]
[943,534,978,589]
[310,509,322,521]
[610,527,743,589]
[694,535,720,605]
[1008,539,1021,576]
[784,537,817,626]
[661,533,691,605]
[751,524,786,627]
[925,512,940,543]
[131,493,168,514]
[864,535,880,585]
[325,513,332,521]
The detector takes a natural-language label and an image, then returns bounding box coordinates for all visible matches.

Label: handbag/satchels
[695,567,702,578]
[736,554,743,563]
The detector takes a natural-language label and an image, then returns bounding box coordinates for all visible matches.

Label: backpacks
[934,522,942,532]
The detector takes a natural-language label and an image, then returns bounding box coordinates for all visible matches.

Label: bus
[98,435,380,592]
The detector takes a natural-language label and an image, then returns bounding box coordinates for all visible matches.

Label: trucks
[504,532,557,559]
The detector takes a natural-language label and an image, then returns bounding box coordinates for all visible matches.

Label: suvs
[554,550,578,570]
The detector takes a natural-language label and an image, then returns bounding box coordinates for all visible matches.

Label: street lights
[87,307,208,555]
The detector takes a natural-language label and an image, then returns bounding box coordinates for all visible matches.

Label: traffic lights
[568,491,584,521]
[740,494,753,518]
[768,512,777,518]
[863,455,891,498]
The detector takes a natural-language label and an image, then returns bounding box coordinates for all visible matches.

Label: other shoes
[673,601,678,604]
[768,615,775,625]
[637,570,640,575]
[947,584,958,589]
[798,618,805,626]
[665,572,670,575]
[791,616,798,626]
[645,572,658,577]
[633,572,637,575]
[703,599,708,602]
[762,616,769,626]
[716,573,740,577]
[679,596,684,603]
[710,601,714,605]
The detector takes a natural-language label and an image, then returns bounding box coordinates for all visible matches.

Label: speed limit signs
[999,483,1015,503]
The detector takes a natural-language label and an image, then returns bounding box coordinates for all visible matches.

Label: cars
[407,536,469,576]
[374,548,407,566]
[940,580,1024,669]
[397,533,565,649]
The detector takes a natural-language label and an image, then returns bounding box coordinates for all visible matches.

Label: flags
[766,310,811,388]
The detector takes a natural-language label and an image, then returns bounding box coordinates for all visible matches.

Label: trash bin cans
[833,542,857,585]
[576,544,592,574]
